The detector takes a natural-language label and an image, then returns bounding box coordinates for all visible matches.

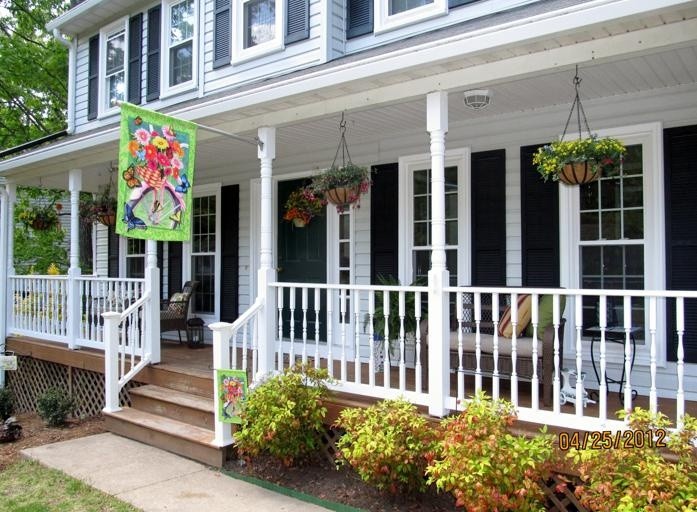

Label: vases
[556,161,602,186]
[96,214,115,226]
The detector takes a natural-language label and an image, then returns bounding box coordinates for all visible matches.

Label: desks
[587,326,643,412]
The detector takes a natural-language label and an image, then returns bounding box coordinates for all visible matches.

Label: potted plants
[19,206,58,232]
[363,271,428,374]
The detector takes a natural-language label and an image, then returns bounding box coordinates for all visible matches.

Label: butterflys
[121,202,148,231]
[167,122,177,137]
[121,163,143,190]
[168,211,185,228]
[175,172,192,195]
[151,200,165,214]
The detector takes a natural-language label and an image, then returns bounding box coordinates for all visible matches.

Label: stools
[187,318,205,348]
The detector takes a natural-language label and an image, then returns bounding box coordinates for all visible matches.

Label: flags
[112,101,199,244]
[214,368,249,426]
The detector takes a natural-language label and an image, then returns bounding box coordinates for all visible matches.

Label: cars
[580,219,642,326]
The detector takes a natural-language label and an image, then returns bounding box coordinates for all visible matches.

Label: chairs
[160,278,199,348]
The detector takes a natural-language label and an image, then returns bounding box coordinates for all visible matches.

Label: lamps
[463,90,494,112]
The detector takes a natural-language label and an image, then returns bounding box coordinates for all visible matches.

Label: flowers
[533,138,625,172]
[283,166,375,228]
[79,193,117,222]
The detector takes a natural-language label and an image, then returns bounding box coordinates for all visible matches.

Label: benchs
[420,302,566,409]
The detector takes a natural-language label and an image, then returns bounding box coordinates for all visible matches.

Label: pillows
[499,286,568,337]
